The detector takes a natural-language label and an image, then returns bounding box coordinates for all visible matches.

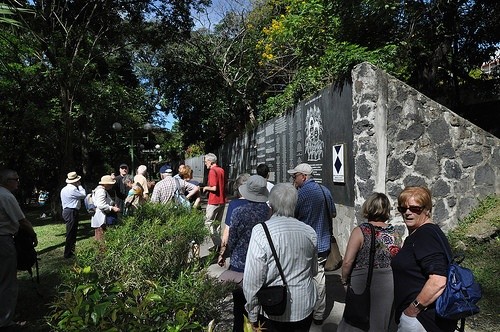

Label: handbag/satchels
[255,284,287,316]
[323,235,343,272]
[218,268,247,298]
[343,284,371,332]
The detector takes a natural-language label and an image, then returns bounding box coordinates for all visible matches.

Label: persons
[150,165,200,208]
[287,163,337,325]
[203,153,224,234]
[227,175,272,332]
[242,181,319,332]
[180,166,200,207]
[60,172,87,261]
[217,174,251,267]
[337,192,402,332]
[122,175,144,216]
[256,163,274,192]
[111,164,133,222]
[0,169,38,329]
[173,164,185,179]
[90,174,120,244]
[390,186,458,332]
[134,166,150,201]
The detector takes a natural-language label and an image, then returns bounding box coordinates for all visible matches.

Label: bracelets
[413,300,427,312]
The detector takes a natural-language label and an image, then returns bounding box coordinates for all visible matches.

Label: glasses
[7,177,19,181]
[398,204,427,214]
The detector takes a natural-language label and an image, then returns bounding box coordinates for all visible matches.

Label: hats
[158,165,173,174]
[120,163,127,168]
[287,163,313,176]
[238,174,270,202]
[98,174,116,185]
[65,171,81,183]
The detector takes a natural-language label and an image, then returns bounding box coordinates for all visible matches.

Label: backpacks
[14,224,40,285]
[420,226,484,319]
[84,190,97,217]
